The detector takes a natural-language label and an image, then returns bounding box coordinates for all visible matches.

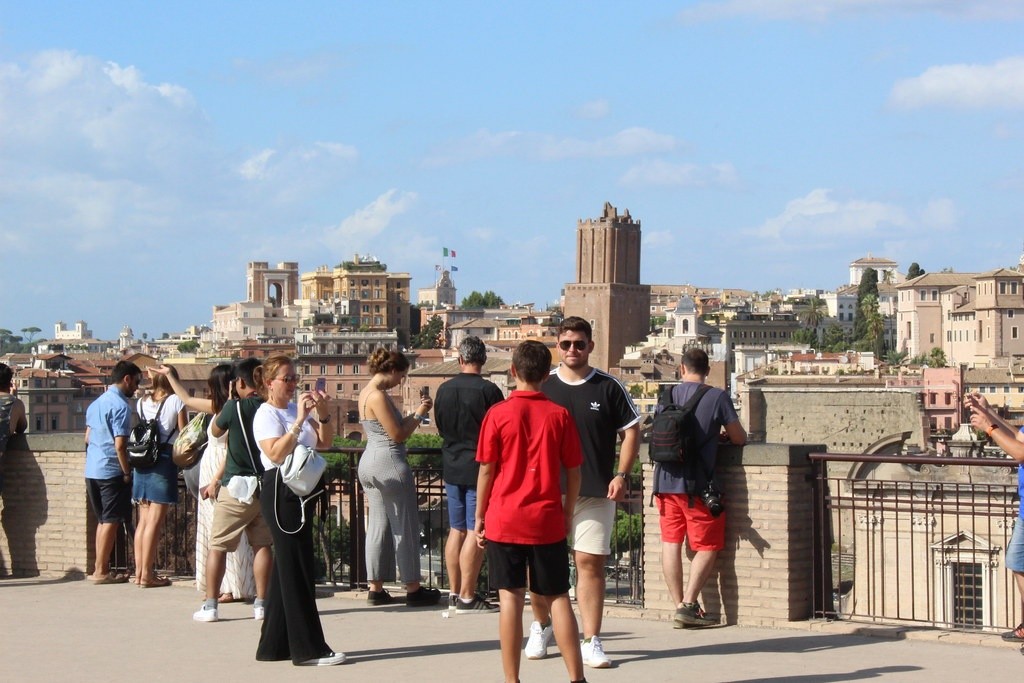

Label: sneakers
[579,636,612,668]
[524,616,553,659]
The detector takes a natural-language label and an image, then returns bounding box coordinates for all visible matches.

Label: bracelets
[987,424,999,437]
[124,473,131,476]
[319,414,331,424]
[213,478,222,485]
[414,413,424,420]
[616,471,631,481]
[289,429,299,438]
[294,423,302,431]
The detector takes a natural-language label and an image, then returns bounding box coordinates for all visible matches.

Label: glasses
[558,340,591,351]
[273,374,300,384]
[136,379,141,387]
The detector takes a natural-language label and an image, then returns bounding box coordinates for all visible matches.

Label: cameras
[701,483,724,515]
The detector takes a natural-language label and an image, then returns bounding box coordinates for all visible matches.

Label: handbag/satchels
[253,474,265,499]
[279,444,327,497]
[171,410,208,470]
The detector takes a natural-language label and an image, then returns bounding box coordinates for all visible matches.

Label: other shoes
[1001,623,1024,642]
[367,589,399,605]
[406,586,441,607]
[193,604,218,622]
[448,595,457,610]
[673,618,686,628]
[202,593,232,602]
[296,651,345,666]
[254,607,264,620]
[456,594,500,614]
[1020,646,1024,655]
[674,600,715,626]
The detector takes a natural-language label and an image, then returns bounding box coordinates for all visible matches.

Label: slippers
[92,572,128,584]
[133,573,169,587]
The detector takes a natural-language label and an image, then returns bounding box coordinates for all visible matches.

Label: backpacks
[126,394,180,467]
[648,384,714,464]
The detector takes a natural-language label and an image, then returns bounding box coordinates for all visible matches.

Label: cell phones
[315,377,325,396]
[422,386,429,396]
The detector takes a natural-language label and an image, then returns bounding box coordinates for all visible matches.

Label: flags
[435,247,458,271]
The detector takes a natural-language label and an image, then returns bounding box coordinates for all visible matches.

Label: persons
[433,336,505,614]
[963,391,1024,655]
[131,364,189,588]
[192,358,274,621]
[652,348,747,629]
[358,348,441,607]
[474,340,587,683]
[253,356,346,666]
[148,364,257,603]
[525,315,642,667]
[84,360,143,584]
[0,363,28,458]
